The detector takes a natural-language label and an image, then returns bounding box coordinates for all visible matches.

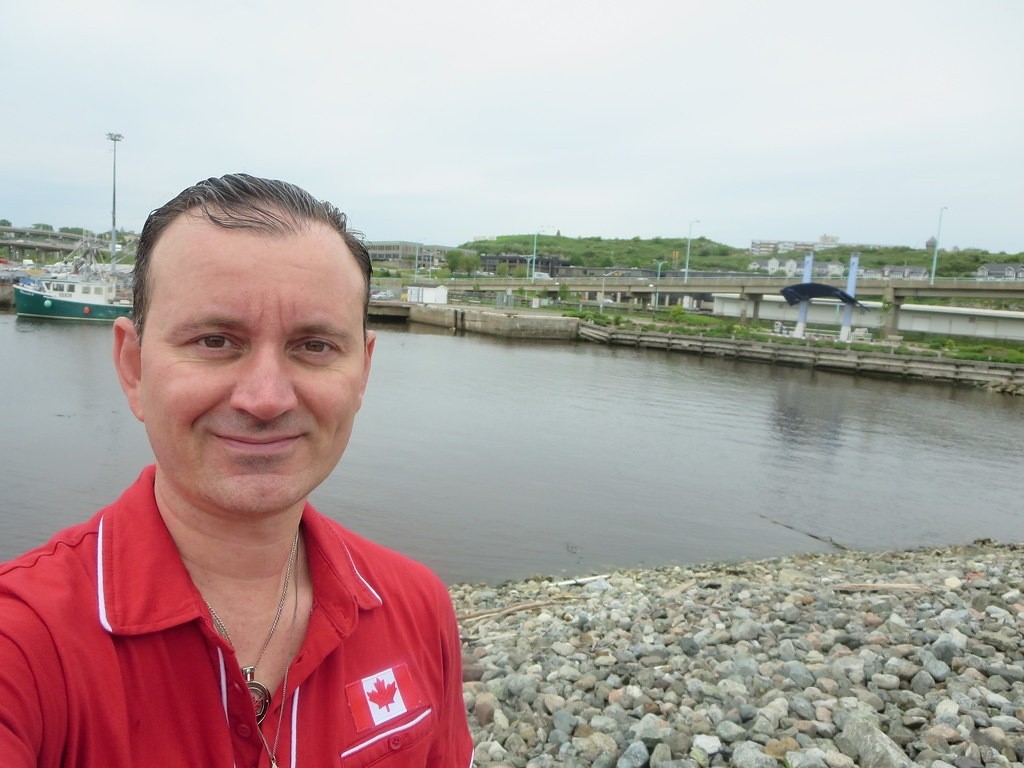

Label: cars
[371,289,393,302]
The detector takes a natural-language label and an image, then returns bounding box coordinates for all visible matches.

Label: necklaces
[210,535,301,768]
[199,526,301,725]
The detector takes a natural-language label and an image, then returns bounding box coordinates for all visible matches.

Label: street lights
[651,257,668,319]
[930,205,949,286]
[683,219,702,284]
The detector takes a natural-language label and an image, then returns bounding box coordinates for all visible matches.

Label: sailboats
[11,131,137,322]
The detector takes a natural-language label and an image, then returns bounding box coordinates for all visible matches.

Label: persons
[0,172,475,768]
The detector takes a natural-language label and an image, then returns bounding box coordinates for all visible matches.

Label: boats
[0,238,101,311]
